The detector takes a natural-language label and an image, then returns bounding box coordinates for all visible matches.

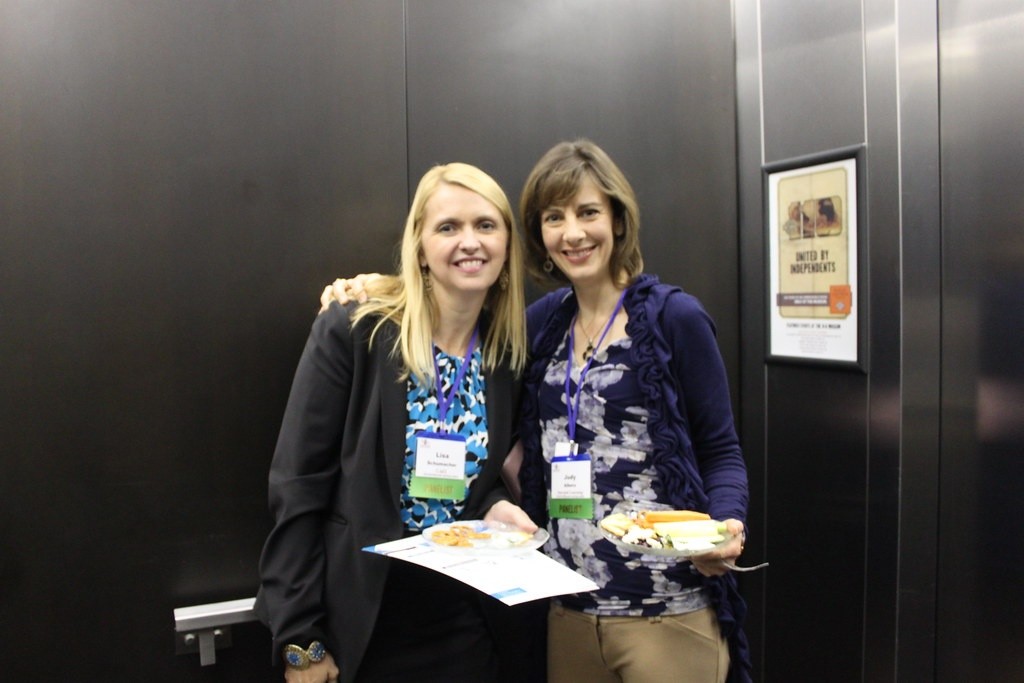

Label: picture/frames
[761,143,867,377]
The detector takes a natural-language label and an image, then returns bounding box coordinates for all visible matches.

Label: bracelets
[281,641,326,670]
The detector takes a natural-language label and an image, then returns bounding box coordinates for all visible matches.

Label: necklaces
[578,308,614,364]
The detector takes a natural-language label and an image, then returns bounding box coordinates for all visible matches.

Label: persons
[255,163,527,683]
[321,138,753,683]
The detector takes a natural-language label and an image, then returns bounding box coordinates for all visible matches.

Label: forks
[691,559,769,571]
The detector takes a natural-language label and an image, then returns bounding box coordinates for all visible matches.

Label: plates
[422,517,552,558]
[597,517,734,556]
